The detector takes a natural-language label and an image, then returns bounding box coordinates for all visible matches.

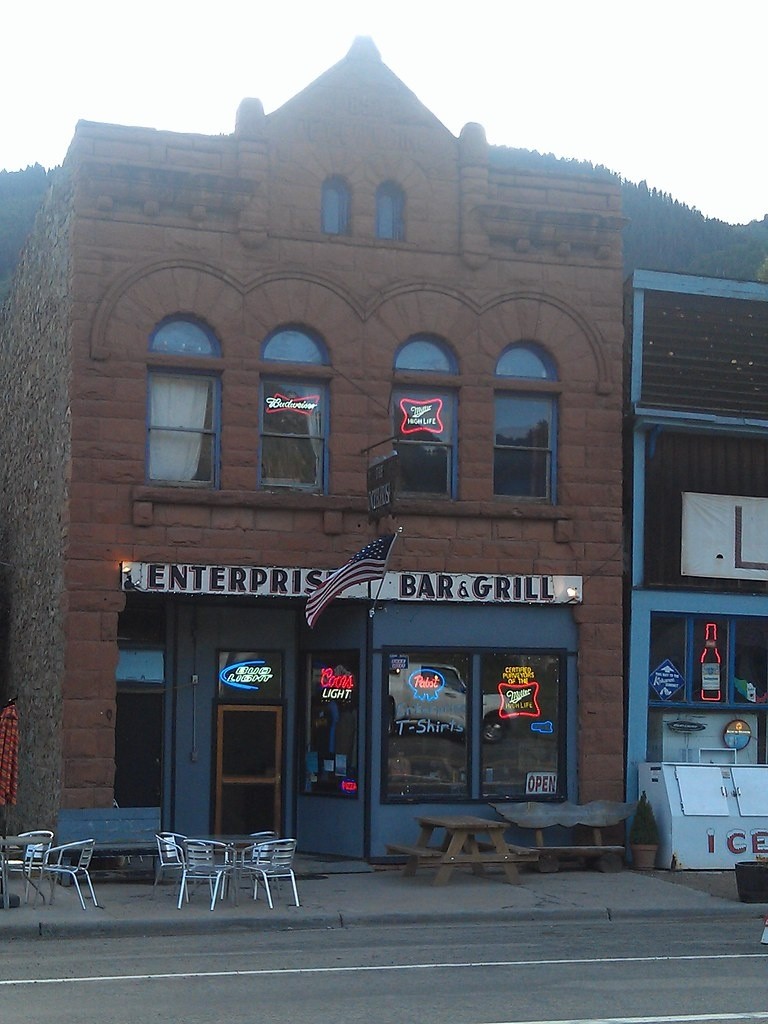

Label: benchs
[54,806,162,886]
[478,840,541,856]
[384,842,441,878]
[488,800,638,873]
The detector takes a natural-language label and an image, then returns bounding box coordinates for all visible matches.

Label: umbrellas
[0,699,19,896]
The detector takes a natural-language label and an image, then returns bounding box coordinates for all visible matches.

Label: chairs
[0,831,55,906]
[177,838,239,913]
[230,830,281,901]
[32,839,98,911]
[240,837,300,909]
[149,832,189,904]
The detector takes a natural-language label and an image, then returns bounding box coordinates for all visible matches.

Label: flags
[305,532,395,629]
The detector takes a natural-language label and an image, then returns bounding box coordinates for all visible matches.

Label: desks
[400,815,521,886]
[213,839,273,902]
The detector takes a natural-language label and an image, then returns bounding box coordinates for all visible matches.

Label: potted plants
[735,853,768,903]
[628,790,659,872]
